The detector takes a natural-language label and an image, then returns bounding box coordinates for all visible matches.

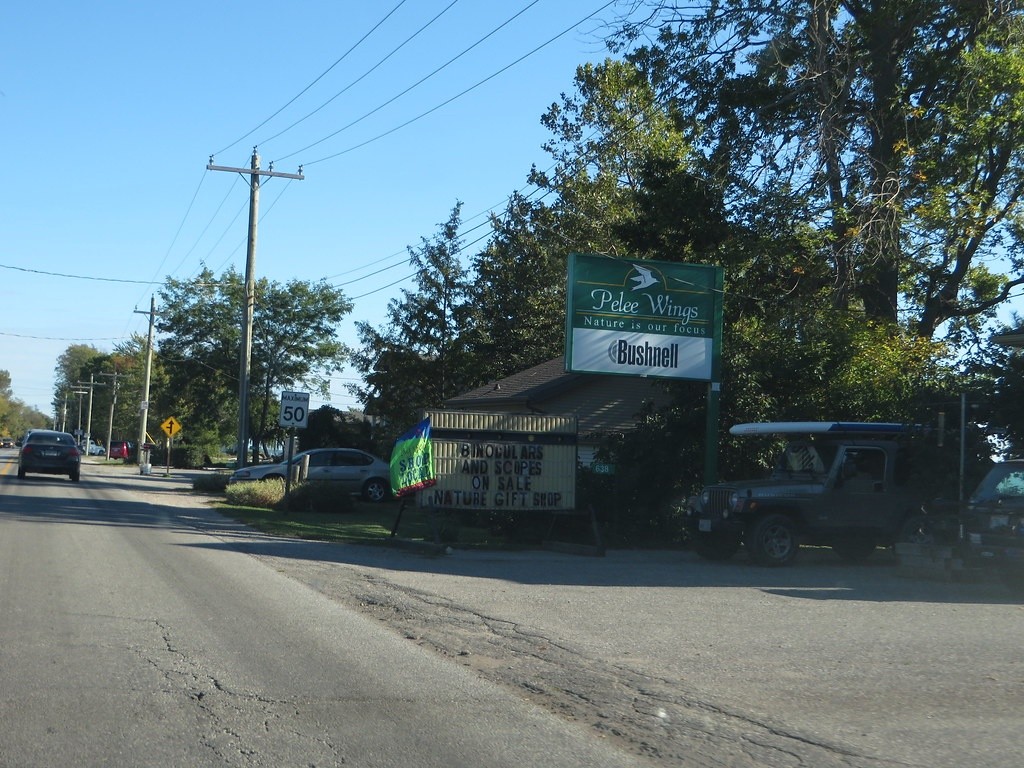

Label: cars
[18,428,82,483]
[110,439,132,461]
[79,439,107,456]
[961,458,1024,581]
[0,436,15,450]
[228,448,392,506]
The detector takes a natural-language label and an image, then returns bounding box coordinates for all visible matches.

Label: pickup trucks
[688,421,974,569]
